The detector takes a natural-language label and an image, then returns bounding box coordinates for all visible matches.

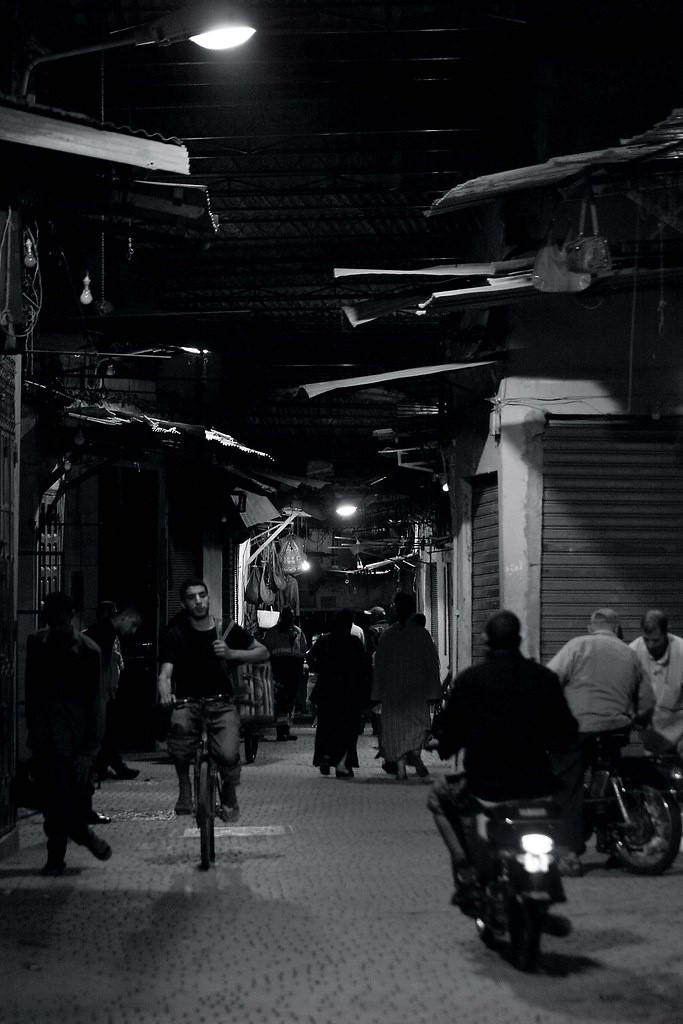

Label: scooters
[577,731,683,875]
[420,739,570,967]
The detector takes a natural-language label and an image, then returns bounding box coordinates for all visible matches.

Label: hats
[363,607,385,616]
[591,607,625,640]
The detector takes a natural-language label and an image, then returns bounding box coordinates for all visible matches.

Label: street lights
[20,4,257,96]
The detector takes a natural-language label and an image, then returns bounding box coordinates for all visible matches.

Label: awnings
[29,376,334,509]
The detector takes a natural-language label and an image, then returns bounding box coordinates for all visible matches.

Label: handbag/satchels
[244,538,304,607]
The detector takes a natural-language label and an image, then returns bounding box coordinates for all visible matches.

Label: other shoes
[396,773,407,780]
[549,858,584,878]
[45,844,65,874]
[174,783,192,815]
[448,881,483,905]
[336,769,355,780]
[277,732,298,742]
[219,785,240,823]
[111,768,140,780]
[411,751,430,778]
[83,833,112,860]
[319,759,330,776]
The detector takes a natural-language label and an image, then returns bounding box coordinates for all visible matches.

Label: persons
[23,591,112,875]
[427,611,595,908]
[546,604,655,877]
[628,608,683,762]
[157,576,271,822]
[266,591,440,780]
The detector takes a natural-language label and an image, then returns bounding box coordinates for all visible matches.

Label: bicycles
[165,692,243,870]
